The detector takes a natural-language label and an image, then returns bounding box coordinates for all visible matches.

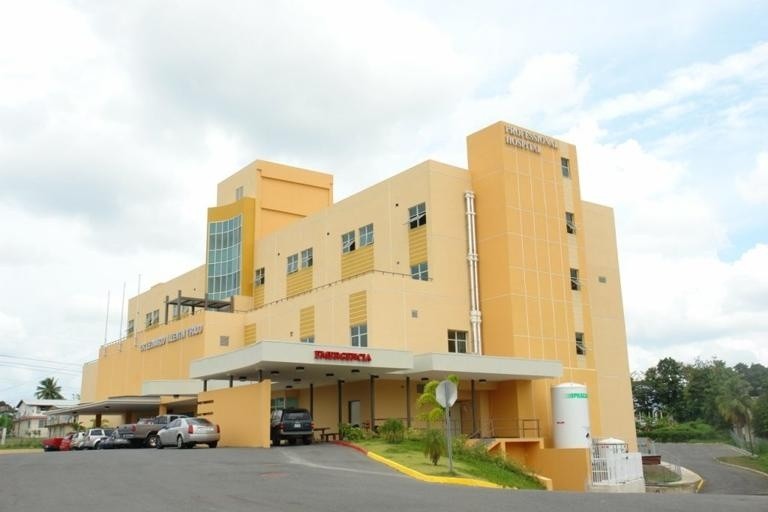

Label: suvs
[270,408,314,445]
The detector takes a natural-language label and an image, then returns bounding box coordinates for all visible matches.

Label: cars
[41,414,220,449]
